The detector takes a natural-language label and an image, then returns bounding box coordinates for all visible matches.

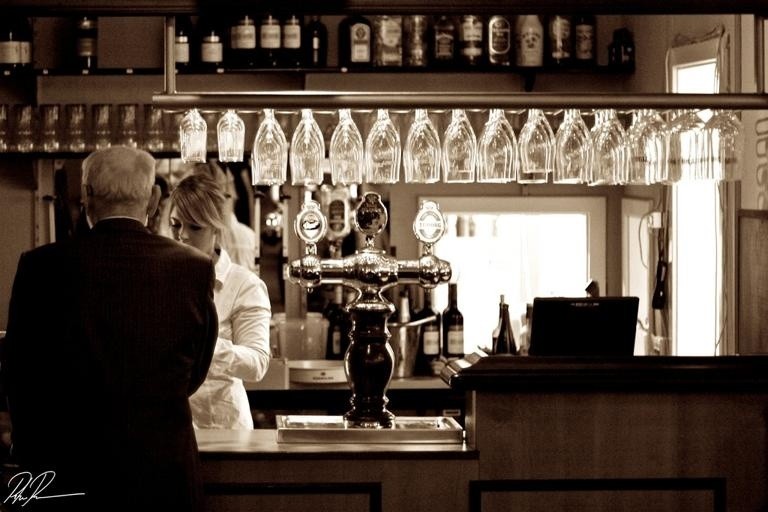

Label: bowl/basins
[280,319,328,359]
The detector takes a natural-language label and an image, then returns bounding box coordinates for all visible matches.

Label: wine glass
[177,105,744,185]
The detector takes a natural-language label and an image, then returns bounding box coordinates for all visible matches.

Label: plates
[287,361,349,384]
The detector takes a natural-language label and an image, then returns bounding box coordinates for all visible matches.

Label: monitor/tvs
[528,296,639,355]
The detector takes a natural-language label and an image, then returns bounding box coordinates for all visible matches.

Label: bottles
[172,13,595,75]
[398,291,412,333]
[443,282,464,358]
[416,288,440,374]
[323,284,346,358]
[1,5,34,77]
[492,303,533,354]
[609,27,635,71]
[70,15,97,73]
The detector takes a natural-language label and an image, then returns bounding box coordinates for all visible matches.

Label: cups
[1,103,182,152]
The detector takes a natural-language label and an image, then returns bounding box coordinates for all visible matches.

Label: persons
[1,145,218,510]
[166,175,273,429]
